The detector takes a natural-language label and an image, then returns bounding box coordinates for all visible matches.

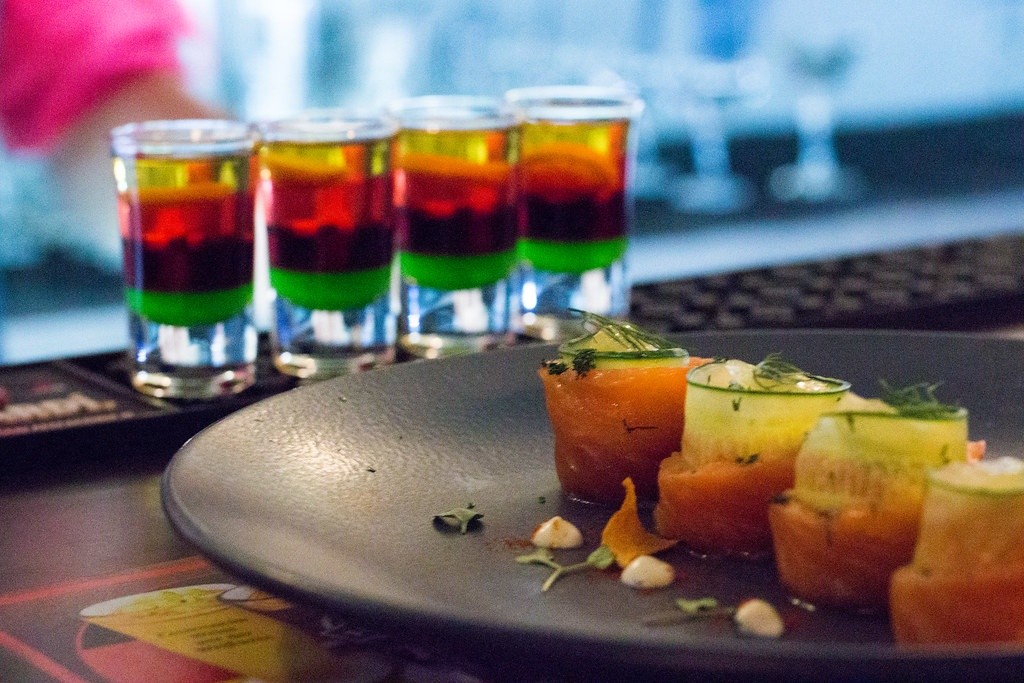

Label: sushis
[543,306,1024,651]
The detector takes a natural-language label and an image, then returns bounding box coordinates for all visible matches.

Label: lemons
[519,143,618,197]
[398,151,511,178]
[263,153,345,186]
[130,182,233,203]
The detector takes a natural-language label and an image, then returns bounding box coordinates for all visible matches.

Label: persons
[0,0,234,187]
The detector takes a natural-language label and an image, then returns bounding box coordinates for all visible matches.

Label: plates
[159,326,1024,683]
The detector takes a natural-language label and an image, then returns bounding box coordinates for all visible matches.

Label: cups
[399,94,520,361]
[108,118,264,401]
[506,85,645,341]
[264,111,403,380]
